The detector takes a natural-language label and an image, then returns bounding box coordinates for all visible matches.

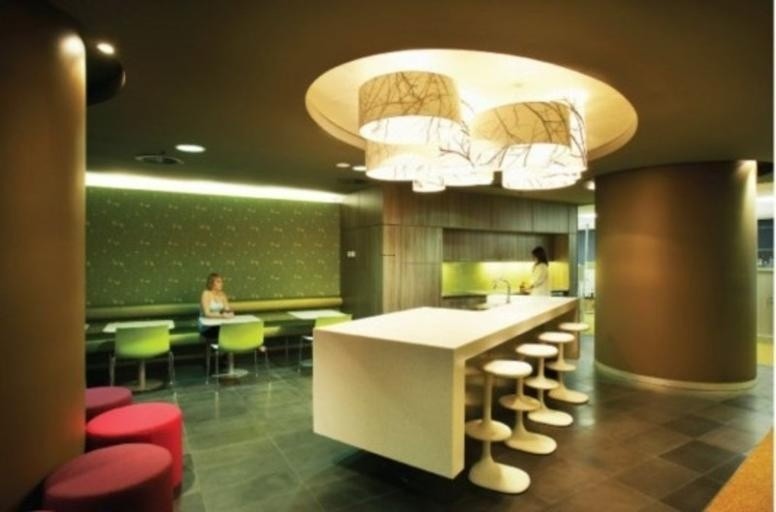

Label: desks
[103,318,176,391]
[197,314,264,381]
[285,306,351,369]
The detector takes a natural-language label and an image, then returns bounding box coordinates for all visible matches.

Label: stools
[525,331,576,428]
[86,401,183,496]
[83,386,132,421]
[465,359,533,496]
[41,442,173,512]
[502,339,560,455]
[547,318,591,408]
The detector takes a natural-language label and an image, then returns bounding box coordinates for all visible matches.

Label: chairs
[296,317,353,374]
[204,321,263,383]
[106,324,172,392]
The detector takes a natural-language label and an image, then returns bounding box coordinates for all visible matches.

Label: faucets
[493,280,511,304]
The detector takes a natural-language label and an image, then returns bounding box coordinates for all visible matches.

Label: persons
[521,246,551,296]
[761,252,773,268]
[198,272,236,376]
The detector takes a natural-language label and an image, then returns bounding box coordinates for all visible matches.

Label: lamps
[357,70,495,194]
[471,100,593,190]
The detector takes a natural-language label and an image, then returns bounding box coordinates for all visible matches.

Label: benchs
[86,297,346,377]
